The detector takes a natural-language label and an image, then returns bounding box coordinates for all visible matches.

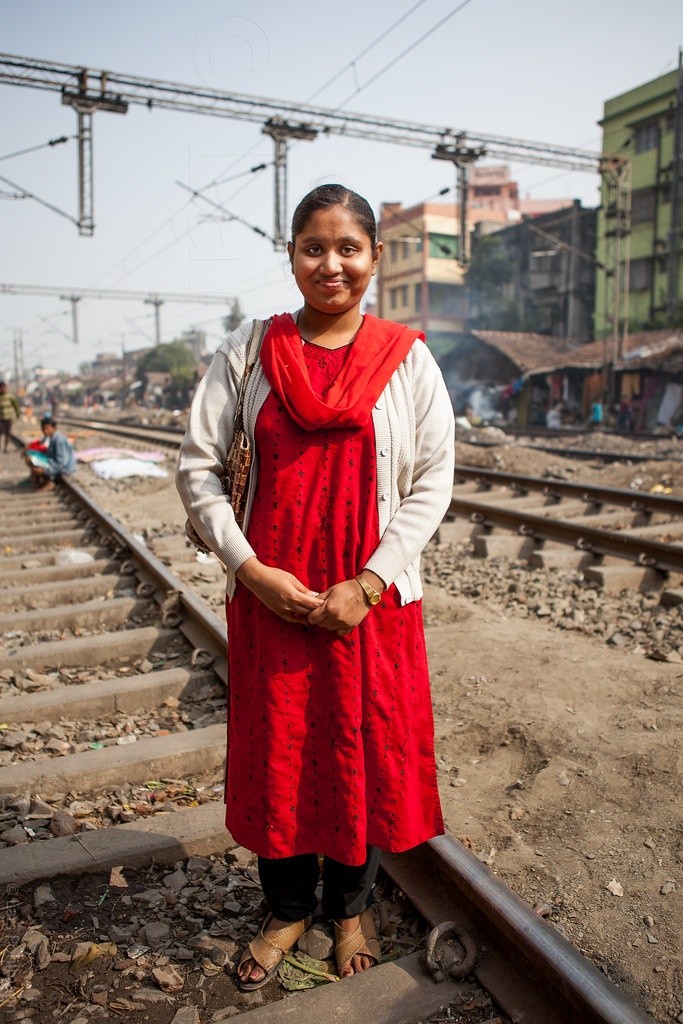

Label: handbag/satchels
[186,317,263,553]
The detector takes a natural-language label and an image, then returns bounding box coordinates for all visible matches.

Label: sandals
[333,906,381,977]
[238,912,312,990]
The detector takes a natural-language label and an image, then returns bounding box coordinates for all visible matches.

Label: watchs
[354,575,381,605]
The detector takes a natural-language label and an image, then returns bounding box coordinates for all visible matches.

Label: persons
[0,381,21,454]
[21,417,76,491]
[175,183,455,991]
[464,405,488,427]
